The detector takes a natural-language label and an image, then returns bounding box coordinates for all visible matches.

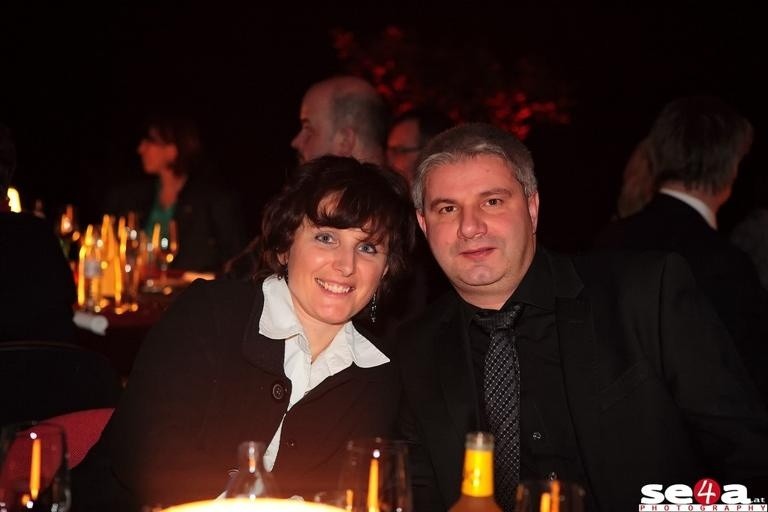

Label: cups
[0,420,72,512]
[334,437,415,511]
[515,478,588,512]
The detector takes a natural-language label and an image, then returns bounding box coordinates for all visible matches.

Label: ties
[475,302,522,511]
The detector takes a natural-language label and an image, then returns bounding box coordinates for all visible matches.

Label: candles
[539,479,560,511]
[369,448,380,512]
[30,430,40,499]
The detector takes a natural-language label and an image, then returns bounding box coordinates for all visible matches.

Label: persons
[2,19,767,512]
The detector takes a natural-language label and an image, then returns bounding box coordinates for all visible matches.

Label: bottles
[444,432,511,512]
[58,202,181,316]
[223,436,283,500]
